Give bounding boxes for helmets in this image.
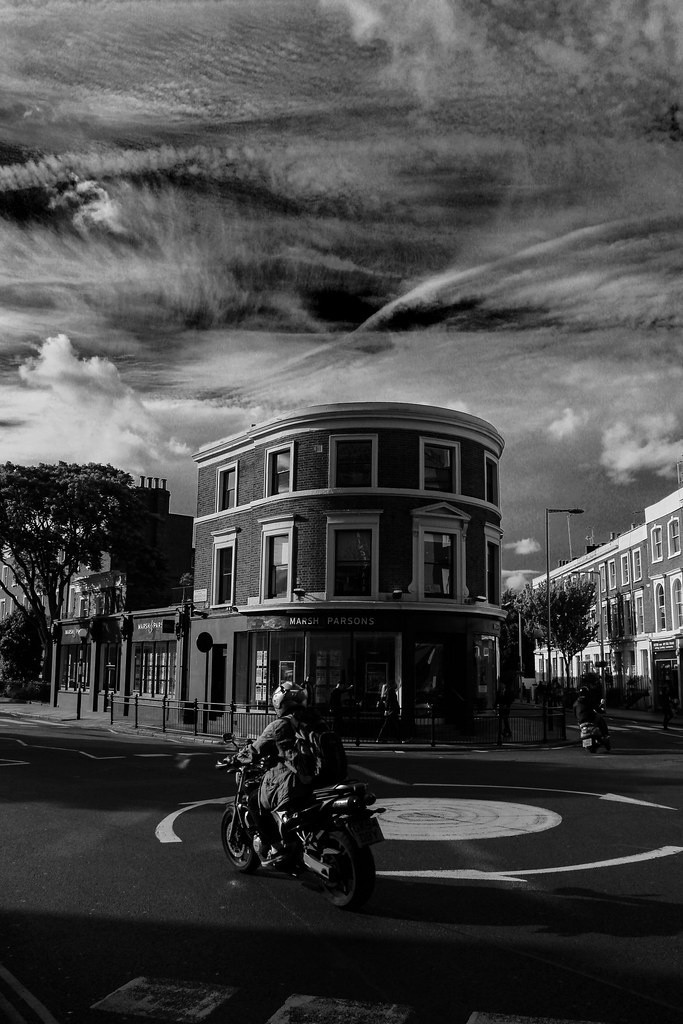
[579,686,589,695]
[272,681,306,718]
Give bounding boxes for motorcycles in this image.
[216,731,380,909]
[581,698,611,754]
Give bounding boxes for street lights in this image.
[542,501,612,709]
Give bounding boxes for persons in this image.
[327,676,572,744]
[572,687,610,738]
[660,685,676,730]
[217,680,349,867]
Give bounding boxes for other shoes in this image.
[402,736,413,744]
[261,850,284,865]
[605,734,611,740]
